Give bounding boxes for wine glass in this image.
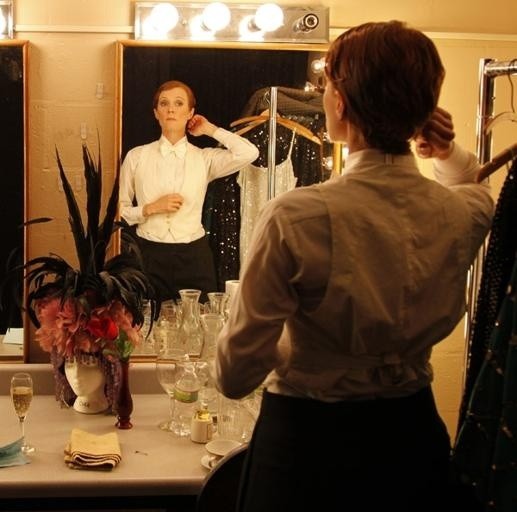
[7,373,35,455]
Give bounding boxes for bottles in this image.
[135,270,255,358]
[132,314,276,472]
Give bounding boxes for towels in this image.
[62,430,121,470]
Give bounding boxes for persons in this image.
[118,80,261,323]
[212,20,495,512]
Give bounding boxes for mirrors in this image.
[113,39,342,361]
[0,41,30,364]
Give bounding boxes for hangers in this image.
[462,57,517,376]
[228,93,322,142]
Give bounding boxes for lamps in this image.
[248,4,284,33]
[149,3,180,33]
[201,3,231,31]
